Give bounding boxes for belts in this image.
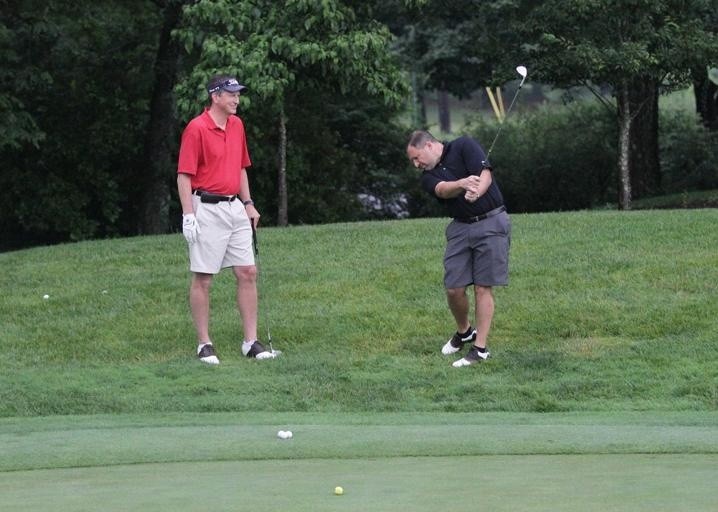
[457,205,507,224]
[192,189,237,201]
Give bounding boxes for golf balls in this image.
[335,486,343,495]
[278,430,293,440]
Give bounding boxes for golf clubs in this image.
[477,64,528,176]
[251,218,281,355]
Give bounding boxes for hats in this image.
[206,77,246,93]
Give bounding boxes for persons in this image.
[177,73,273,364]
[403,130,511,368]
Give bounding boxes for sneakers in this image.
[241,339,275,359]
[197,341,219,365]
[452,346,491,367]
[441,327,477,356]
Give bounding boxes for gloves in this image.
[182,213,202,245]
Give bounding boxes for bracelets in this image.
[244,200,254,206]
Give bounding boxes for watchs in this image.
[476,194,480,199]
